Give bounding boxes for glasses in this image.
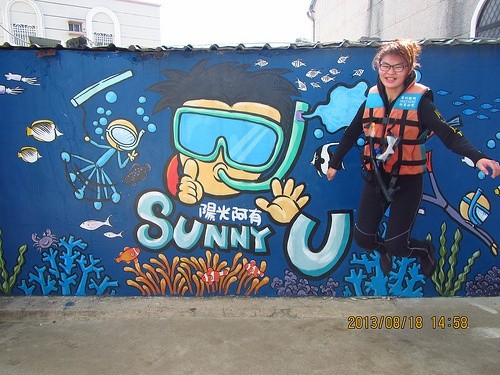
[378,61,410,73]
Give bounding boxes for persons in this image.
[325,41,500,277]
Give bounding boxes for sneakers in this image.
[378,238,393,276]
[419,241,436,276]
